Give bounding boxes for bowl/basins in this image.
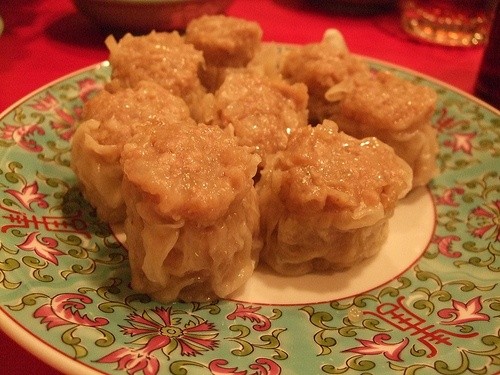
[71,0,236,36]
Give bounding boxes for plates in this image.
[0,43,500,374]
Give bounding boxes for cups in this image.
[397,0,497,49]
